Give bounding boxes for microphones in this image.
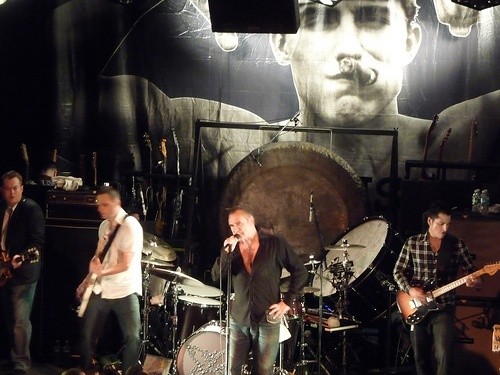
[323,257,337,272]
[290,110,301,121]
[309,194,314,222]
[225,234,240,249]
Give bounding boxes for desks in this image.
[40,187,102,240]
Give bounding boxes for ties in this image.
[9,209,14,220]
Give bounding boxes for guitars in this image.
[396,261,500,325]
[20,129,183,240]
[77,256,100,318]
[1,246,39,287]
[421,112,478,177]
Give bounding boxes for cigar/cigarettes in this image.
[339,57,377,85]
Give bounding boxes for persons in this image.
[56,0,500,185]
[0,171,45,375]
[155,215,167,234]
[76,190,143,375]
[212,207,308,375]
[393,208,481,375]
[36,162,57,185]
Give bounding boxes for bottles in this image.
[472,189,489,214]
[492,325,500,352]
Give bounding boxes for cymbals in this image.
[325,244,366,250]
[141,254,176,267]
[142,231,177,262]
[281,287,320,293]
[148,267,206,288]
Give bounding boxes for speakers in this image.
[38,215,122,362]
[446,214,500,375]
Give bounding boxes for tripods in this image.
[291,247,362,375]
[117,264,178,375]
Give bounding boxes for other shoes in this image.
[13,369,29,375]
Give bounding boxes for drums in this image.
[163,281,226,352]
[289,295,305,316]
[273,315,302,375]
[175,321,254,375]
[308,214,406,326]
